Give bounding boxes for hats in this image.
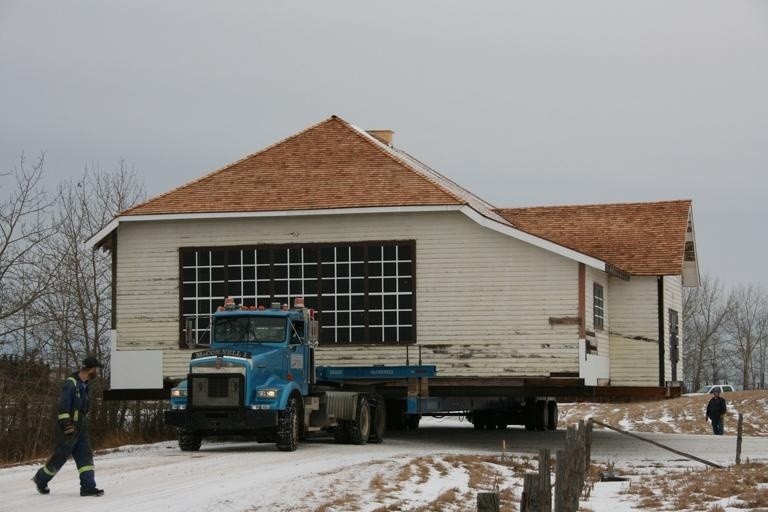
[81,357,104,368]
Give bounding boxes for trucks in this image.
[164,298,585,453]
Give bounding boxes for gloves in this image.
[63,420,74,437]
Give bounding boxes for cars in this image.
[679,385,734,397]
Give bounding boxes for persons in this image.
[32,356,105,497]
[705,390,726,435]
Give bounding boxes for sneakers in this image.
[33,475,49,494]
[80,488,104,496]
